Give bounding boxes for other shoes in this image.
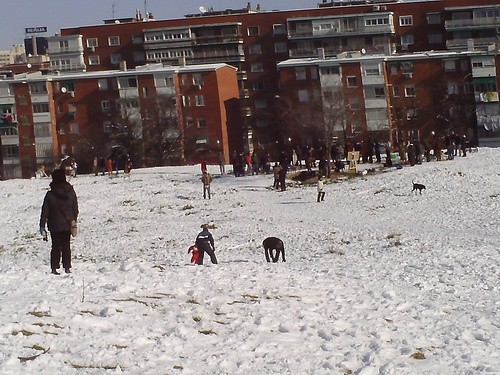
[52,271,60,275]
[65,268,72,273]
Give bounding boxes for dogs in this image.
[412,178,426,195]
[263,237,286,262]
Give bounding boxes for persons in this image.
[200,159,207,174]
[187,241,205,264]
[195,224,218,265]
[93,154,132,177]
[263,237,286,263]
[39,168,80,275]
[231,124,473,192]
[201,172,212,200]
[317,175,326,202]
[218,151,226,175]
[37,158,79,179]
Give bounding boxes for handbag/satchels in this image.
[71,219,77,237]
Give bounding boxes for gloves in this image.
[40,226,47,235]
